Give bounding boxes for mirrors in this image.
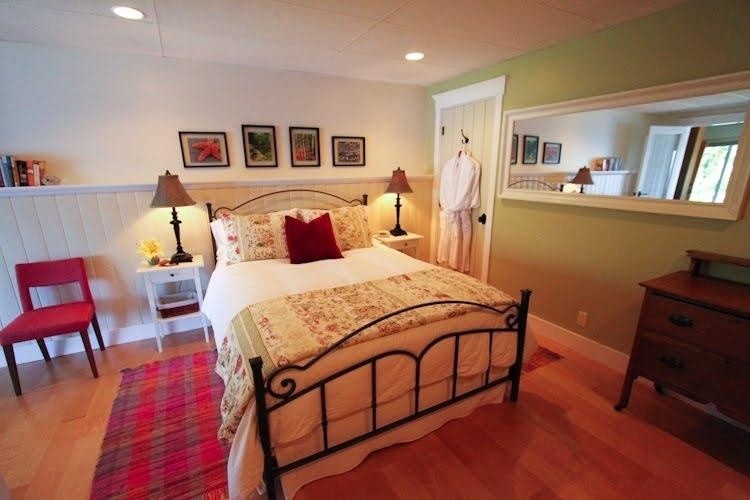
[497,70,750,222]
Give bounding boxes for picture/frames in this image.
[331,136,366,166]
[287,126,321,167]
[510,134,518,164]
[543,141,562,164]
[523,135,540,164]
[240,124,278,168]
[177,131,230,168]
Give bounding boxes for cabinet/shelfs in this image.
[614,250,750,427]
[508,170,640,196]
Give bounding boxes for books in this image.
[593,157,622,172]
[0,156,47,188]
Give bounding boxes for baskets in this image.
[155,291,199,318]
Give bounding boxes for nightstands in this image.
[372,233,423,259]
[136,255,209,354]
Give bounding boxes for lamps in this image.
[385,167,415,236]
[150,170,198,264]
[568,165,596,195]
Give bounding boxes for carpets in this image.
[89,344,567,500]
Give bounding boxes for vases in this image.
[146,255,159,264]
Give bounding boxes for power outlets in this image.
[576,310,589,329]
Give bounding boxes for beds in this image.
[204,189,533,500]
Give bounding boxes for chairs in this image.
[1,257,106,396]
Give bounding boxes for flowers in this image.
[136,239,161,262]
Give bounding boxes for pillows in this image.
[218,207,298,267]
[297,204,373,252]
[284,212,345,264]
